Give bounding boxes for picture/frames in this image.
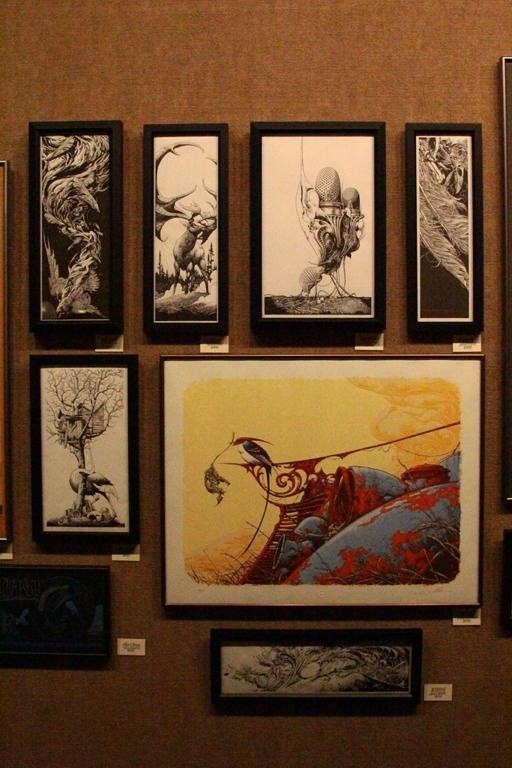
[0,563,111,664]
[143,124,229,334]
[159,354,484,607]
[501,56,512,505]
[29,353,140,545]
[405,122,483,338]
[0,160,11,543]
[501,528,512,637]
[250,120,386,332]
[210,629,423,706]
[29,120,123,335]
